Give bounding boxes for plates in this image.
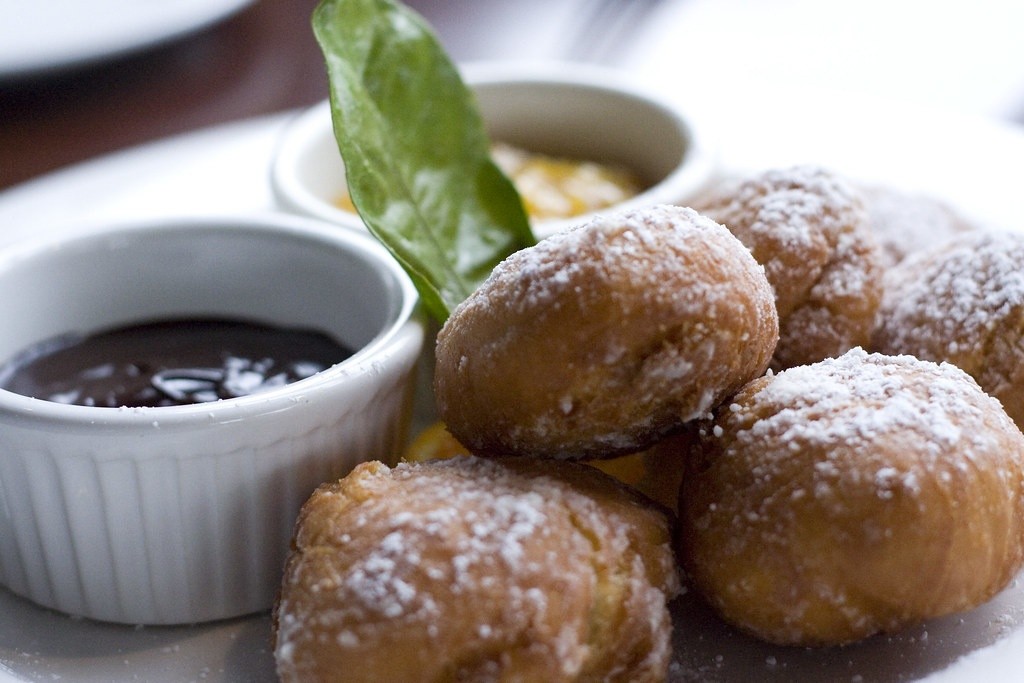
[0,105,1023,683]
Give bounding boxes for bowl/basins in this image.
[1,205,437,628]
[270,63,710,240]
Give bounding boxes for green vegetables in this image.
[314,2,535,323]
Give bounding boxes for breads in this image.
[275,165,1024,683]
